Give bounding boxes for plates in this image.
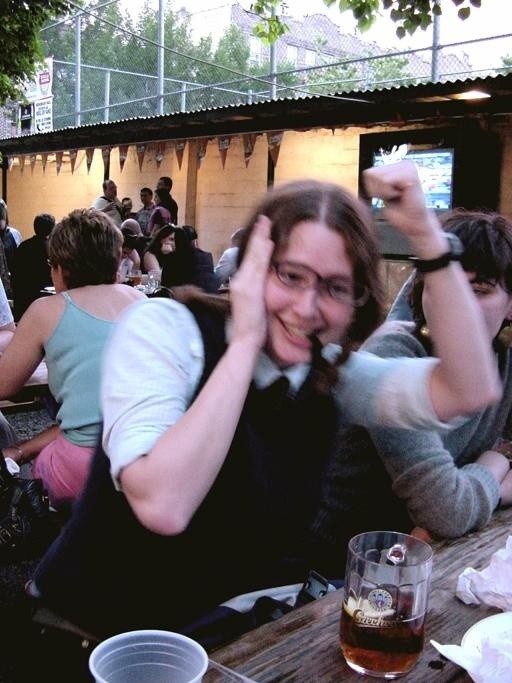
[458,611,512,682]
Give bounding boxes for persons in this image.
[214,229,244,283]
[0,198,23,293]
[95,177,178,235]
[10,155,504,676]
[10,213,55,302]
[307,209,512,570]
[118,207,220,292]
[2,207,149,505]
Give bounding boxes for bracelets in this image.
[413,233,461,271]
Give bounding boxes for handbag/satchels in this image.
[0,477,61,564]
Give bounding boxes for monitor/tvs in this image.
[369,148,455,220]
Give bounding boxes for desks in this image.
[203,509,511,683]
[1,355,49,415]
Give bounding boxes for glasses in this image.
[272,257,369,305]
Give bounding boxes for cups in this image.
[339,529,436,677]
[89,628,209,683]
[129,271,162,295]
